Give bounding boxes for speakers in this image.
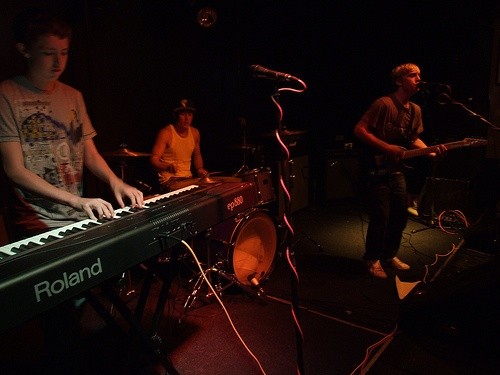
[268,155,308,214]
[426,176,474,223]
[324,154,363,200]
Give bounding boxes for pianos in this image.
[0,183,259,375]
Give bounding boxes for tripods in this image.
[181,230,243,310]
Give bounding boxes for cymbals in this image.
[99,148,151,156]
[169,173,242,191]
[263,129,307,135]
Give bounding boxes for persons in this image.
[0,19,144,375]
[149,98,210,192]
[353,64,448,280]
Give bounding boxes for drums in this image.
[205,206,279,293]
[238,166,276,205]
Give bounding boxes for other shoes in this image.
[390,257,410,270]
[366,258,388,279]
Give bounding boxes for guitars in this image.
[372,134,489,189]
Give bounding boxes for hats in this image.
[169,95,197,114]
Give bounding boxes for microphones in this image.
[248,275,266,294]
[419,81,448,89]
[250,64,297,83]
